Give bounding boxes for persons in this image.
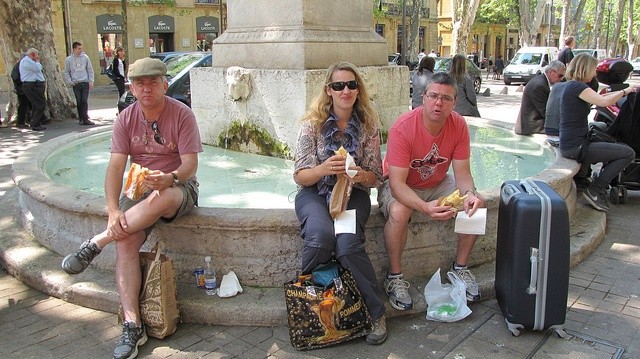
[292,61,389,346]
[112,46,126,99]
[495,54,505,80]
[11,52,51,129]
[105,40,110,51]
[448,54,481,117]
[487,54,493,72]
[558,35,576,66]
[428,50,437,57]
[559,52,636,212]
[63,42,95,125]
[515,61,566,135]
[418,48,426,64]
[19,48,46,131]
[412,56,436,110]
[61,56,203,358]
[105,45,112,61]
[377,72,486,312]
[544,82,568,135]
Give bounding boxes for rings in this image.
[332,167,333,170]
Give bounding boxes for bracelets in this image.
[464,190,475,195]
[622,89,626,97]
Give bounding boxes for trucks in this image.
[571,48,606,61]
[503,47,560,85]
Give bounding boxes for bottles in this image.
[203,255,217,295]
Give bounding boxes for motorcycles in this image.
[594,57,640,127]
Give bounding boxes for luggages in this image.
[495,180,570,338]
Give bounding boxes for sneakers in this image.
[366,315,387,344]
[113,320,147,359]
[446,261,481,301]
[582,184,610,212]
[84,120,95,125]
[62,238,101,274]
[383,270,414,311]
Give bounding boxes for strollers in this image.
[591,89,640,205]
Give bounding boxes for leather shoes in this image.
[32,125,46,129]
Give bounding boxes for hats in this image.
[127,57,167,78]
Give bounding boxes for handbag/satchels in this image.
[284,252,375,351]
[118,242,177,339]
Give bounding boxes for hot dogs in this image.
[334,144,349,157]
[440,190,467,210]
[141,167,159,178]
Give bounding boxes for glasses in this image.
[553,69,564,78]
[329,81,358,91]
[152,120,164,144]
[425,94,453,103]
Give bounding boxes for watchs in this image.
[170,172,179,188]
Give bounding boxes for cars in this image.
[118,51,212,114]
[149,52,183,63]
[467,55,488,69]
[630,57,640,75]
[388,52,401,66]
[410,56,483,97]
